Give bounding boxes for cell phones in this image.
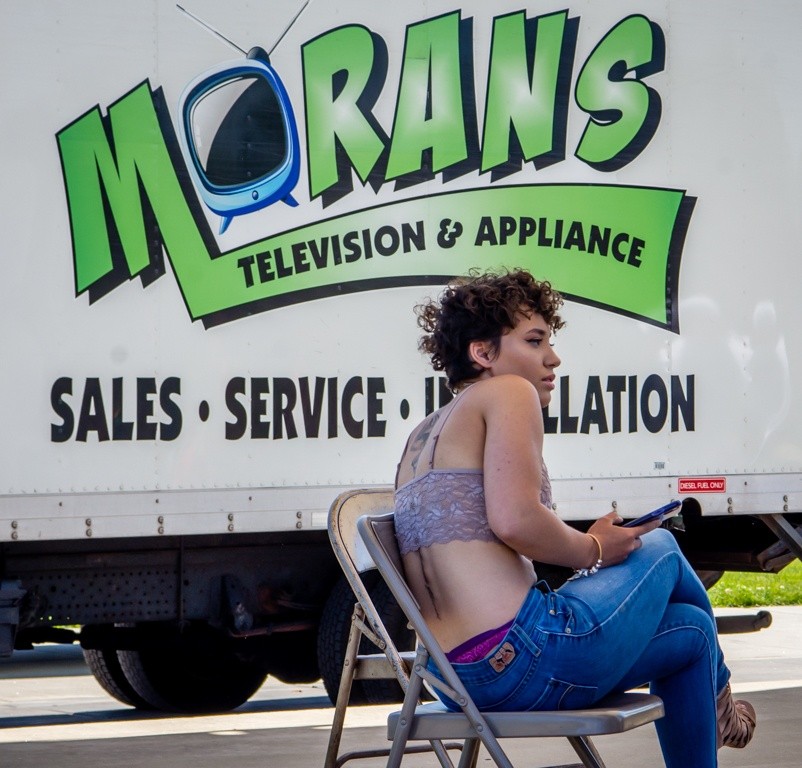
[623,500,682,528]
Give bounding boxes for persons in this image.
[392,265,756,768]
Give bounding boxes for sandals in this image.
[717,682,756,752]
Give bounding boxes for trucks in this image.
[1,0,802,720]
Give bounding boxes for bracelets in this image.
[573,533,603,577]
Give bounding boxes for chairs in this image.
[356,511,665,768]
[324,490,456,768]
